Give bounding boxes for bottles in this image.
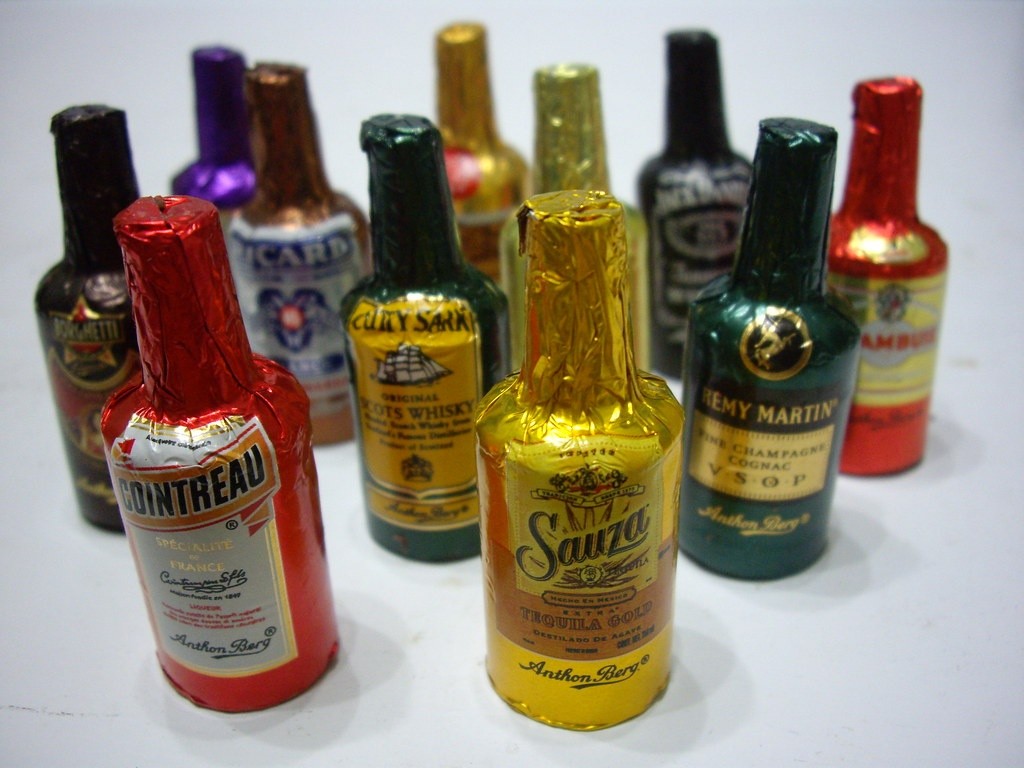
[227,61,372,448]
[432,25,526,291]
[472,190,685,732]
[100,195,337,713]
[677,118,862,581]
[171,47,257,233]
[35,103,143,534]
[338,112,509,566]
[635,30,754,382]
[823,79,948,477]
[497,62,649,377]
